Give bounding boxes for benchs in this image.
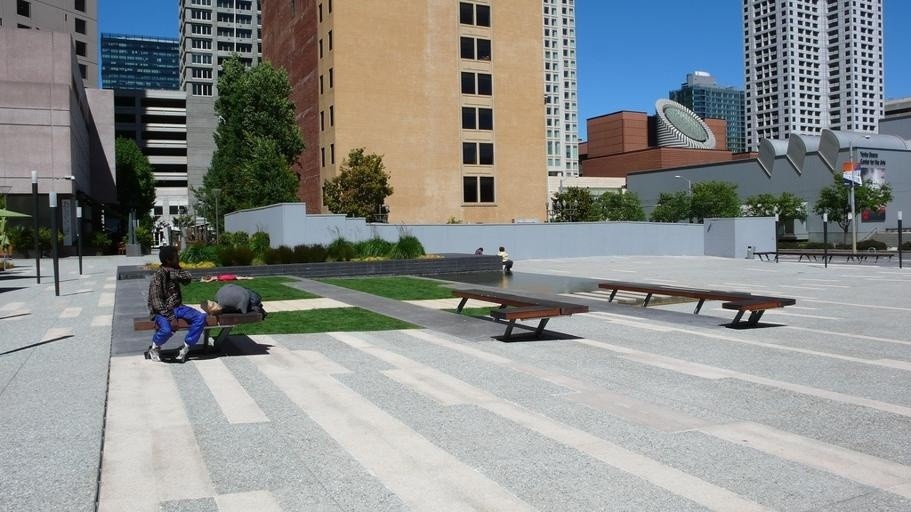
[452,289,589,341]
[133,310,263,359]
[599,282,796,326]
[753,251,899,264]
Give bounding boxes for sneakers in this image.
[175,347,189,363]
[148,346,161,362]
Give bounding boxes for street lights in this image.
[76,207,83,275]
[49,191,59,297]
[31,171,40,284]
[849,135,871,253]
[824,214,827,268]
[676,175,692,194]
[898,211,902,268]
